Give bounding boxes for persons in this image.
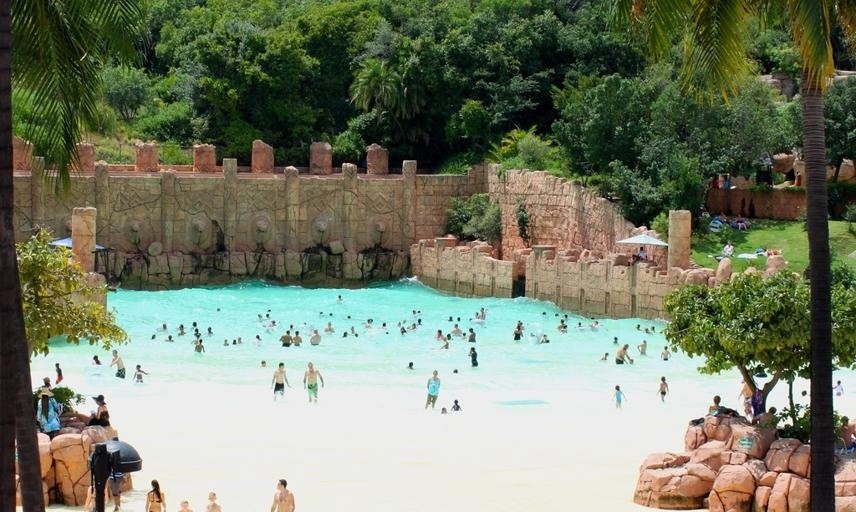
[637,246,647,260]
[708,171,802,257]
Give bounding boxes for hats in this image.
[38,387,55,398]
[92,395,107,405]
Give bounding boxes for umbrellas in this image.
[51,237,104,251]
[618,234,668,251]
